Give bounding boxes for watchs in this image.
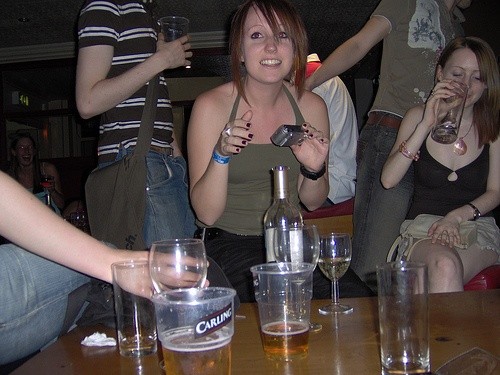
[465,202,481,221]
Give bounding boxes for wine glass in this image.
[315,232,355,315]
[273,224,323,334]
[147,238,207,370]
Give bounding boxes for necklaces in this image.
[452,118,477,158]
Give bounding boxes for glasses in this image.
[16,144,34,151]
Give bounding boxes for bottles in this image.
[45,194,55,212]
[262,165,307,322]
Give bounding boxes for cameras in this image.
[270,125,307,147]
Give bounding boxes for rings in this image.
[224,127,232,136]
[431,88,436,95]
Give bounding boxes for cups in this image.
[111,259,158,358]
[157,15,190,43]
[151,287,236,375]
[376,262,432,375]
[431,80,468,144]
[70,209,88,230]
[250,262,315,363]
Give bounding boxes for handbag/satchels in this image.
[84,154,147,252]
[57,255,241,338]
[386,213,477,263]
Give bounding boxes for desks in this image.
[6,287,500,375]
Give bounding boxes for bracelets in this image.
[398,138,421,162]
[209,145,232,165]
[299,161,327,181]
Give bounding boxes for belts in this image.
[150,144,174,157]
[194,229,265,241]
[366,112,401,128]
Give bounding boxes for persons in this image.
[284,50,360,206]
[4,132,66,212]
[303,0,471,295]
[186,0,379,303]
[0,170,210,366]
[73,0,200,253]
[380,35,500,295]
